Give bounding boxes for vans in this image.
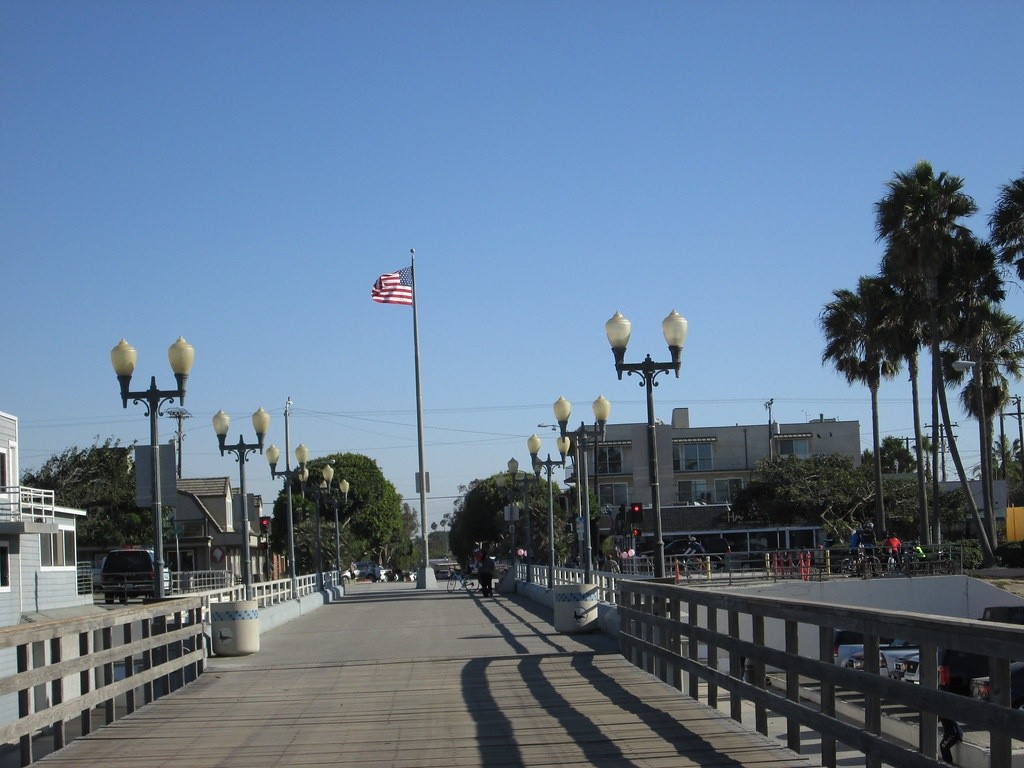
[341,560,381,583]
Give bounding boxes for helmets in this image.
[889,533,897,538]
[867,522,875,530]
[856,526,864,532]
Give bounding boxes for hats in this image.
[607,554,612,559]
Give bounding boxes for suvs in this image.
[101,548,174,605]
[637,537,732,575]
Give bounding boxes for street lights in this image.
[553,394,611,587]
[507,457,544,581]
[299,465,334,593]
[604,310,689,683]
[527,433,571,588]
[110,335,196,706]
[266,442,309,600]
[213,405,270,600]
[319,478,351,588]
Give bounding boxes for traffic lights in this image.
[300,504,310,522]
[631,502,644,524]
[259,516,270,535]
[633,528,642,536]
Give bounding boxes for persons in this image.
[263,559,274,581]
[348,562,356,586]
[609,545,621,557]
[913,541,926,561]
[884,533,905,567]
[938,717,963,765]
[832,522,877,577]
[476,550,494,597]
[701,493,711,503]
[603,555,621,573]
[684,537,705,562]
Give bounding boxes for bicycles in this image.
[840,548,942,580]
[936,546,956,575]
[446,566,480,593]
[672,554,715,577]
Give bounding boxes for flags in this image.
[371,265,412,306]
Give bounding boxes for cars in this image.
[437,569,452,581]
[378,567,418,582]
[831,628,1024,711]
